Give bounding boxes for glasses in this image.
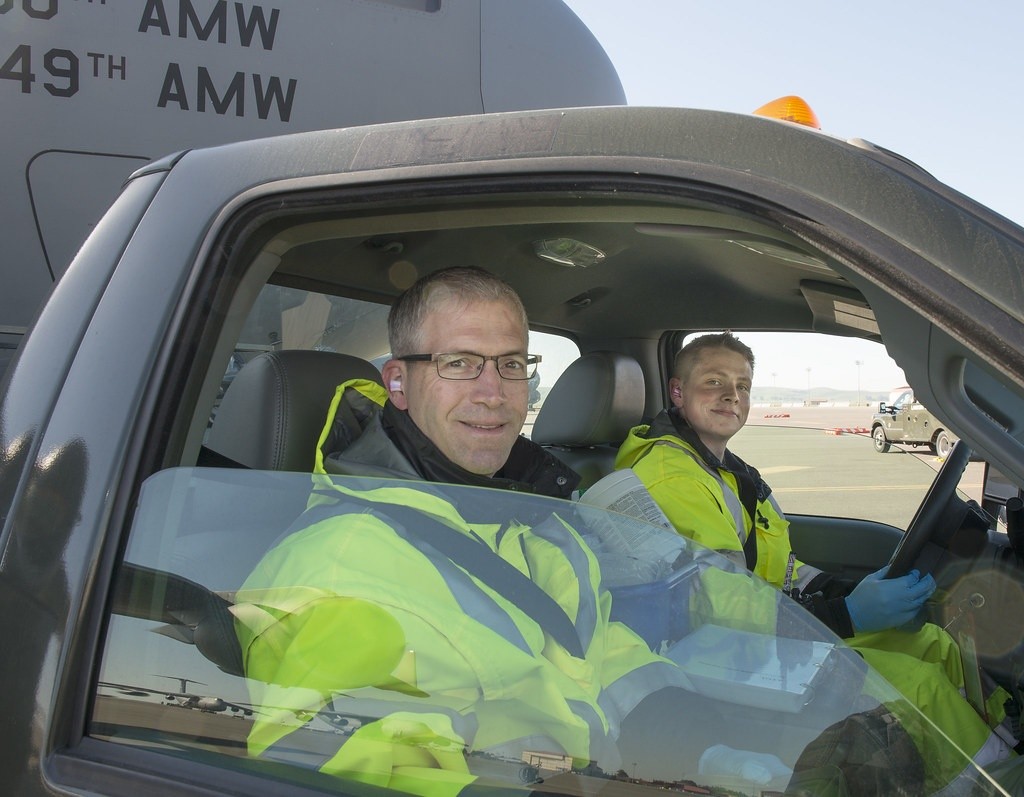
[396,352,542,380]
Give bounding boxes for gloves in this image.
[698,744,794,785]
[845,566,937,633]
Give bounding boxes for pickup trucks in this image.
[870,386,961,461]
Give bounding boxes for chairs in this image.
[531,352,645,490]
[205,350,385,473]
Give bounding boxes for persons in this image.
[229,265,794,797]
[610,329,1024,796]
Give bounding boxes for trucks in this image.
[1,0,1024,796]
